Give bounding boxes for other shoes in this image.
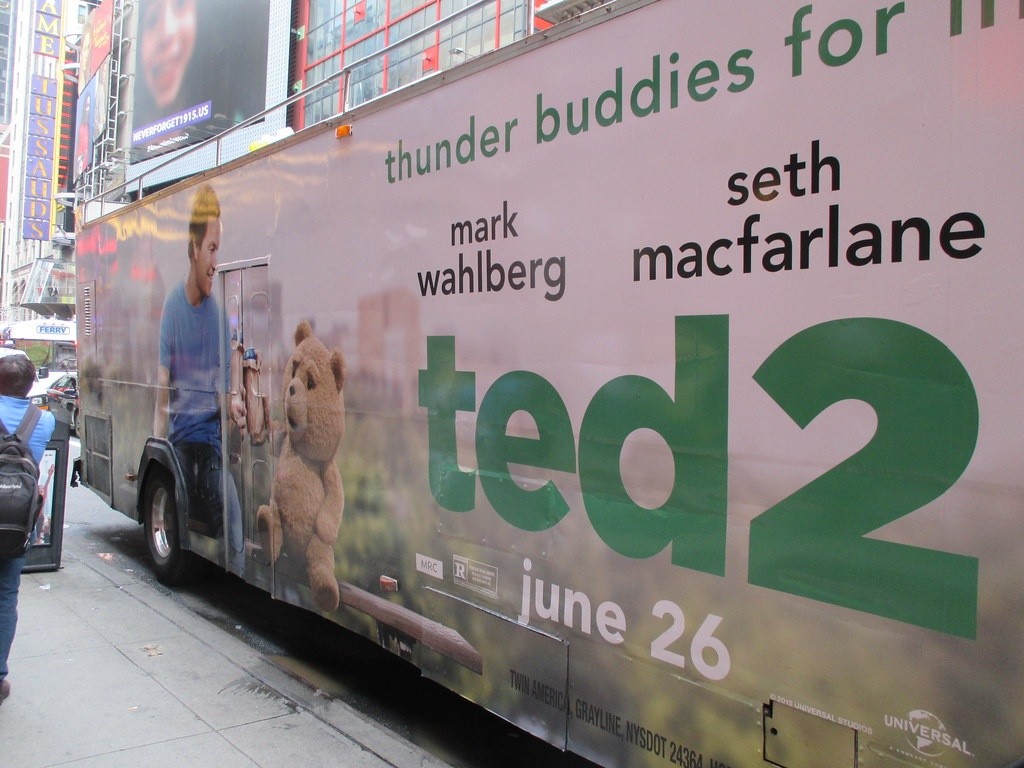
[0,679,10,704]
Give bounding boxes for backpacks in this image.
[0,397,43,562]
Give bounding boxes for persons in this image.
[154,185,247,580]
[0,355,55,704]
[134,0,220,162]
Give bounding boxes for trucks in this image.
[70,0,1023,768]
[0,312,78,411]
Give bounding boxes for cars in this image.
[46,374,81,439]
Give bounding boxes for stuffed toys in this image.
[257,320,347,613]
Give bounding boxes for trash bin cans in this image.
[20,394,72,574]
[68,359,77,370]
